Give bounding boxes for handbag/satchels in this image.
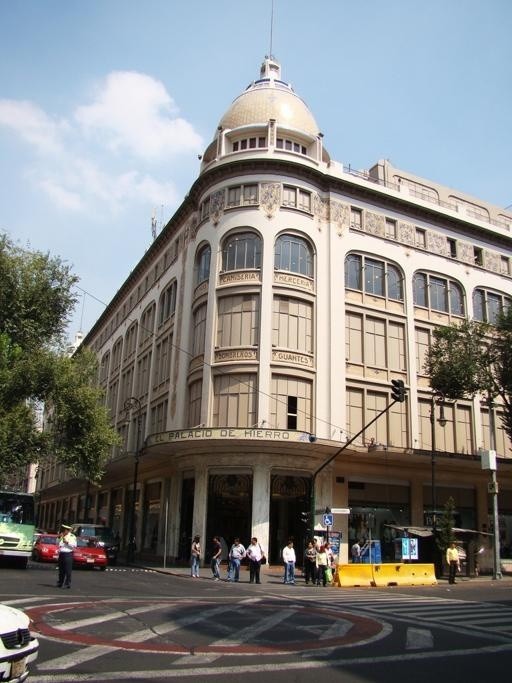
[324,568,335,584]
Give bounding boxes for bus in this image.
[1,489,40,569]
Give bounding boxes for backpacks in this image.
[261,556,267,566]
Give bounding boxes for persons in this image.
[302,537,338,587]
[350,538,360,563]
[191,534,201,578]
[282,540,295,584]
[225,536,245,583]
[207,536,222,581]
[445,541,460,584]
[56,523,78,590]
[246,537,265,584]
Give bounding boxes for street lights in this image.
[35,465,45,528]
[479,390,503,580]
[430,391,449,579]
[121,397,141,562]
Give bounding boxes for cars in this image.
[1,603,40,682]
[33,523,119,571]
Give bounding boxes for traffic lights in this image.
[390,378,403,402]
[300,511,310,530]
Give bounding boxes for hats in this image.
[61,523,73,531]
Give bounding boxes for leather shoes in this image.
[447,581,458,584]
[192,575,200,577]
